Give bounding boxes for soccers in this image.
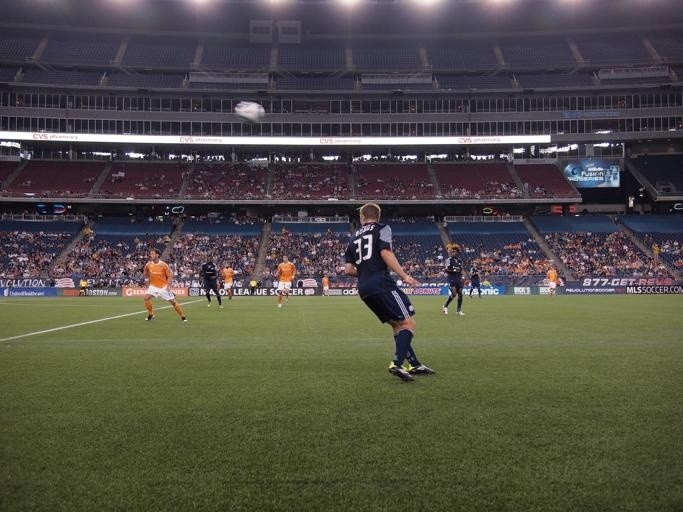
[234,100,266,123]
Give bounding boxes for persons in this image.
[79,279,88,296]
[143,249,187,322]
[321,275,329,297]
[248,278,257,296]
[546,266,557,295]
[221,264,235,300]
[199,254,224,309]
[345,202,436,382]
[273,255,296,308]
[442,248,465,316]
[469,262,482,299]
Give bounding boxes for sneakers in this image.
[145,314,155,321]
[442,305,448,314]
[208,299,212,307]
[277,304,282,308]
[219,305,223,308]
[182,317,188,322]
[286,300,288,303]
[389,360,435,381]
[456,311,465,315]
